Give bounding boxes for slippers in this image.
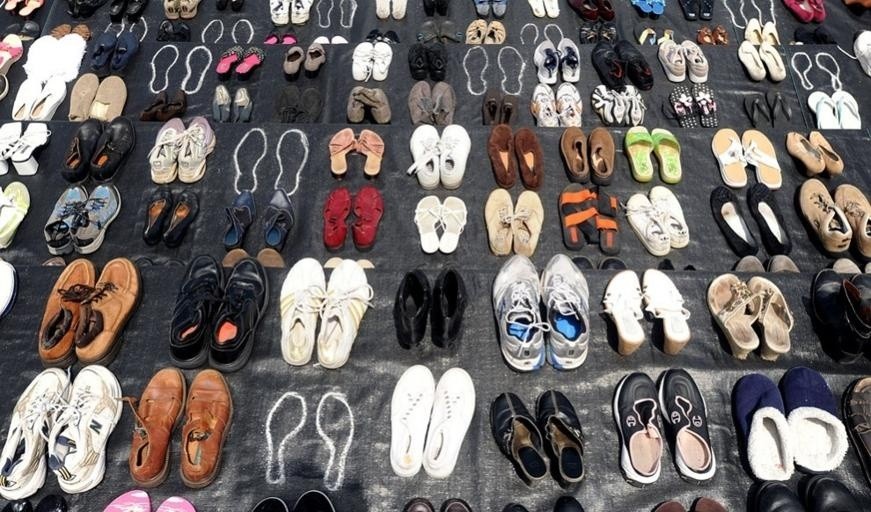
[216,45,244,79]
[235,46,264,79]
[18,0,44,21]
[465,19,487,45]
[669,84,699,127]
[328,127,356,176]
[383,30,400,44]
[580,21,598,43]
[366,28,382,44]
[741,129,783,190]
[357,129,384,179]
[711,128,748,188]
[691,83,720,128]
[18,20,39,41]
[0,33,23,75]
[270,0,290,26]
[11,80,40,122]
[30,74,67,122]
[290,0,313,25]
[474,0,490,18]
[416,21,439,43]
[4,0,22,15]
[630,0,652,18]
[173,22,190,42]
[633,21,657,45]
[700,0,714,20]
[491,0,507,19]
[651,128,682,184]
[108,0,128,23]
[413,194,441,256]
[438,196,468,254]
[778,366,848,476]
[679,0,700,20]
[0,23,20,40]
[156,19,173,42]
[624,125,654,183]
[263,188,294,251]
[731,373,795,484]
[440,20,462,44]
[646,0,666,20]
[832,90,862,129]
[126,0,147,20]
[808,90,841,129]
[766,88,792,128]
[744,93,772,128]
[598,21,618,44]
[68,73,99,121]
[89,75,127,122]
[558,182,620,255]
[483,20,506,44]
[657,24,675,45]
[222,190,255,251]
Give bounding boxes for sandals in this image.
[72,24,91,41]
[4,122,51,176]
[372,42,392,82]
[843,377,871,480]
[0,122,21,176]
[51,23,71,39]
[710,186,759,257]
[351,42,374,83]
[746,182,793,254]
[352,185,385,253]
[322,187,352,254]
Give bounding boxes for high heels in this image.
[536,390,585,490]
[283,46,305,82]
[786,131,825,177]
[138,91,168,122]
[109,31,139,78]
[642,267,691,356]
[747,276,794,362]
[490,392,548,489]
[155,89,186,120]
[809,131,844,179]
[280,26,297,45]
[89,30,116,77]
[707,273,763,360]
[303,43,325,79]
[602,270,643,356]
[263,27,281,45]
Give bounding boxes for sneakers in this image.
[212,85,231,122]
[163,0,180,20]
[178,116,216,184]
[428,42,447,82]
[834,183,871,260]
[533,39,559,85]
[0,181,30,250]
[388,364,435,477]
[279,257,326,366]
[483,88,501,124]
[439,124,471,190]
[626,193,671,257]
[619,84,646,126]
[179,0,201,19]
[271,85,301,124]
[783,0,813,24]
[543,0,560,18]
[528,0,546,17]
[431,82,456,125]
[74,256,142,367]
[591,39,628,87]
[38,258,99,370]
[614,40,654,91]
[561,125,589,184]
[316,259,370,371]
[422,367,476,480]
[656,366,716,485]
[587,127,614,186]
[500,95,518,125]
[539,254,590,370]
[410,124,440,190]
[555,81,583,127]
[0,366,71,502]
[407,80,433,124]
[232,87,252,124]
[531,82,559,128]
[853,30,871,77]
[658,39,686,83]
[649,186,690,249]
[808,0,826,24]
[296,88,323,123]
[612,371,664,489]
[514,128,544,191]
[591,84,625,126]
[557,38,581,83]
[758,41,787,82]
[492,253,546,372]
[513,191,544,256]
[680,40,709,84]
[44,182,89,256]
[799,178,853,258]
[47,363,122,495]
[484,188,514,257]
[69,182,122,254]
[737,40,766,82]
[408,43,427,79]
[149,117,186,184]
[488,123,515,188]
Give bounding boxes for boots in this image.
[348,86,380,124]
[812,268,871,365]
[394,268,430,349]
[851,273,871,304]
[354,87,392,123]
[431,266,468,350]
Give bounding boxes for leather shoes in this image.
[697,23,715,45]
[2,498,32,512]
[572,256,597,270]
[439,498,471,512]
[231,0,244,12]
[115,367,187,488]
[757,480,807,512]
[592,0,616,23]
[142,182,173,244]
[762,21,781,45]
[168,253,226,369]
[403,498,434,512]
[713,24,729,46]
[567,1,599,22]
[179,368,233,489]
[60,117,105,185]
[216,0,228,10]
[89,115,136,187]
[161,185,200,249]
[599,257,628,270]
[67,0,80,17]
[804,472,859,512]
[744,18,764,45]
[33,494,67,512]
[77,0,106,17]
[208,255,269,373]
